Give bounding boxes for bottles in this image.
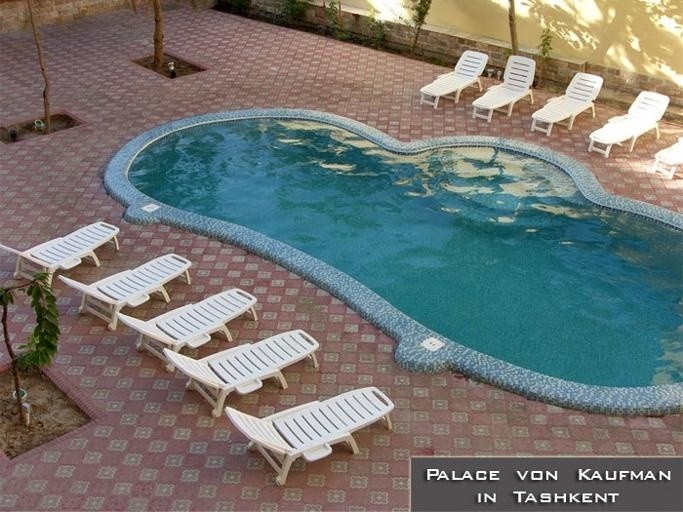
[7,128,16,142]
[167,62,176,79]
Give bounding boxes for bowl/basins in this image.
[34,119,43,130]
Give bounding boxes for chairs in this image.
[530,71,605,137]
[652,140,683,180]
[115,288,258,374]
[471,55,537,124]
[419,49,489,110]
[57,253,192,332]
[162,329,320,419]
[223,385,395,487]
[0,221,120,290]
[588,91,671,159]
